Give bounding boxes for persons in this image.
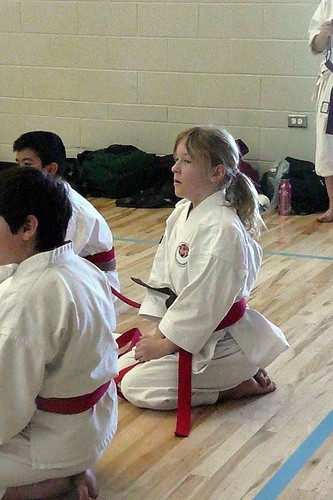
[114,125,289,412]
[307,0,333,223]
[0,130,120,500]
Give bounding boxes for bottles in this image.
[278,179,292,216]
[266,168,277,180]
